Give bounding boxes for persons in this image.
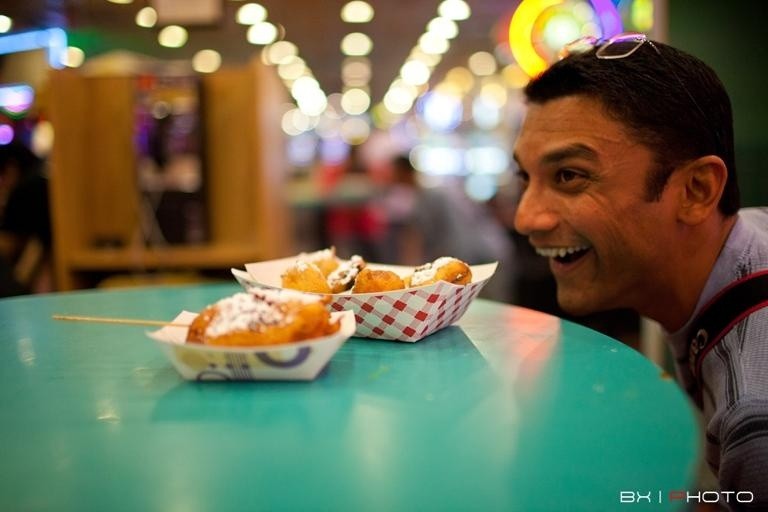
[1,118,54,297]
[386,156,516,303]
[511,36,766,511]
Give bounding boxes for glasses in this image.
[564,31,706,120]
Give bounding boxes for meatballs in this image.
[186,290,341,346]
[283,248,472,292]
